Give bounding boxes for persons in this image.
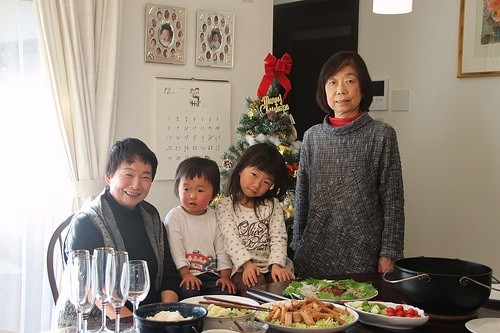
[51,137,180,330]
[164,144,295,298]
[149,9,231,62]
[290,52,405,279]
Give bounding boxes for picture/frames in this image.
[144,3,188,64]
[456,0,500,79]
[196,9,235,68]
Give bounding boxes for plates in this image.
[254,299,359,333]
[343,301,430,330]
[291,279,379,305]
[465,318,500,333]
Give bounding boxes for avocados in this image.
[354,300,388,315]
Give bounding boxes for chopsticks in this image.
[198,296,274,312]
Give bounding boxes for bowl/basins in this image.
[178,294,260,330]
[134,303,208,333]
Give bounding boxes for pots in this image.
[382,256,500,316]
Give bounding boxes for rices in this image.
[146,310,194,321]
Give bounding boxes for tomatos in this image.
[385,305,422,317]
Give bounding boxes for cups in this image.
[230,321,269,333]
[200,329,239,333]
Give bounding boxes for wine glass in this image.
[64,248,150,333]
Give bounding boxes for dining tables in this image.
[78,273,477,333]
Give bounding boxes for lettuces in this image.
[283,277,377,300]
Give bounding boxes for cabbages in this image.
[260,309,355,329]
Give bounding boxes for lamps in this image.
[371,0,412,15]
